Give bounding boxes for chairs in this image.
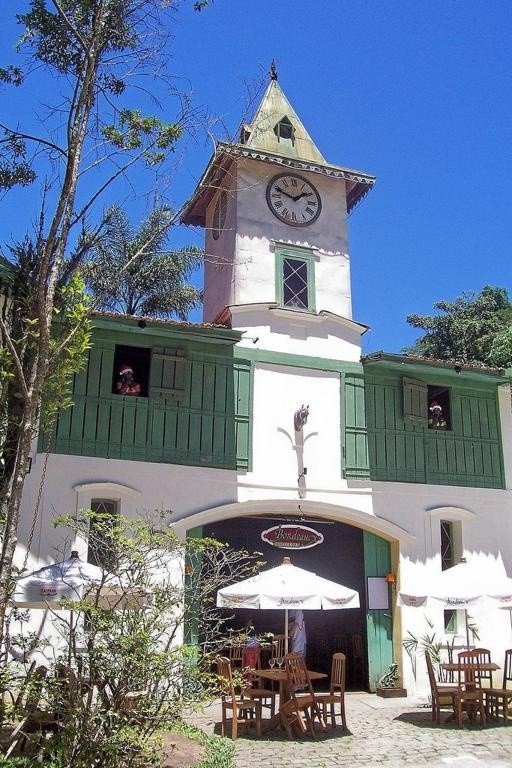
[425,647,512,729]
[216,632,368,741]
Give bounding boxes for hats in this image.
[119,365,134,375]
[429,400,441,411]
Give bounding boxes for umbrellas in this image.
[10,552,157,670]
[217,557,361,670]
[396,559,512,681]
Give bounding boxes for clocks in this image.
[266,172,322,228]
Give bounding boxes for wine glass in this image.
[268,659,275,672]
[276,658,283,673]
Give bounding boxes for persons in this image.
[427,401,447,430]
[115,365,141,397]
[285,609,310,692]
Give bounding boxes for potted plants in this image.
[376,662,407,699]
